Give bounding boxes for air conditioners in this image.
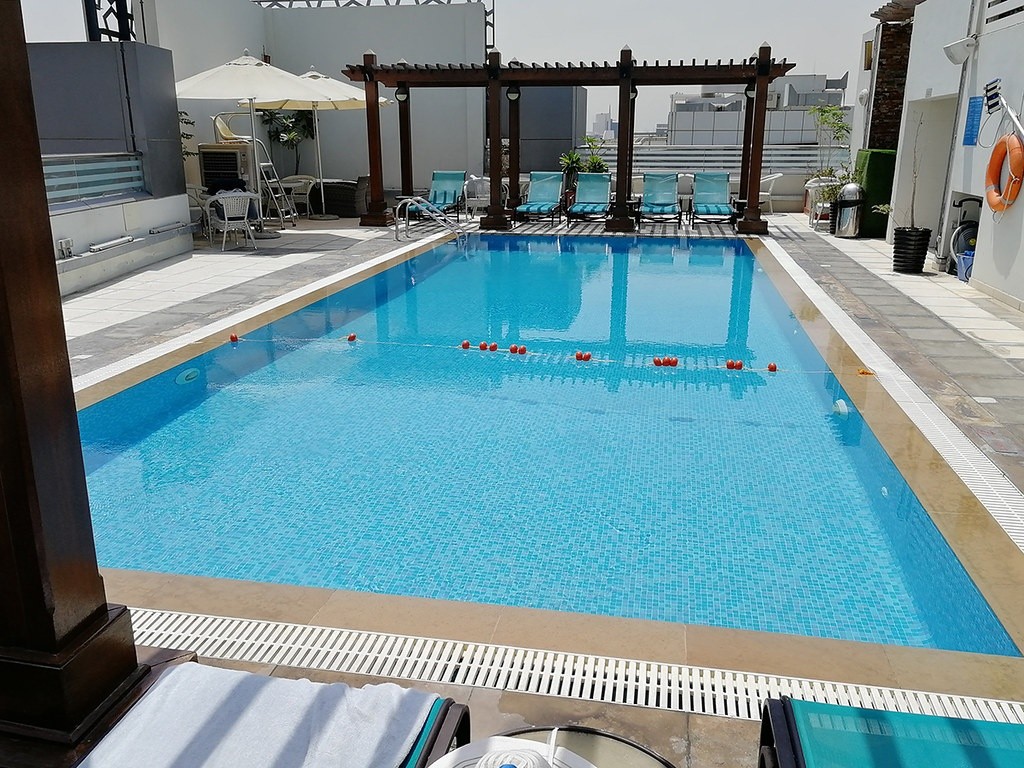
[766,93,777,108]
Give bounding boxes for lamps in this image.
[745,84,755,98]
[395,86,408,101]
[506,86,521,101]
[631,85,638,100]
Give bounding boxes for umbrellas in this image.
[174,47,357,240]
[238,64,395,220]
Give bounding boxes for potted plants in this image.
[802,104,852,220]
[558,136,608,208]
[871,112,933,273]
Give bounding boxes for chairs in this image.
[409,171,467,222]
[686,171,739,230]
[566,172,611,228]
[501,170,566,227]
[639,172,683,230]
[464,177,490,223]
[759,172,783,215]
[188,173,317,251]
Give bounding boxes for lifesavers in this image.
[985,131,1024,211]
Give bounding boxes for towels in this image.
[76,661,441,768]
